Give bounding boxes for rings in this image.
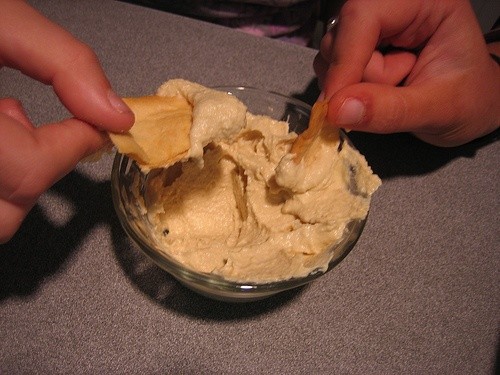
[324,14,339,33]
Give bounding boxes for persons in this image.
[0,2,133,247]
[314,1,500,148]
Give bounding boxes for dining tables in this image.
[0,0,500,375]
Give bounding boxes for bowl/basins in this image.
[110,83,371,301]
